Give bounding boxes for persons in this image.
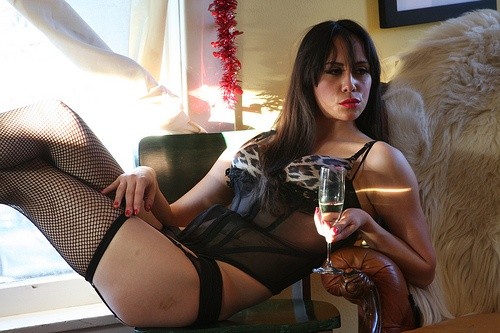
[0,19,437,330]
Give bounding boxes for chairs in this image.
[132,131,340,333]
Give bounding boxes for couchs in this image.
[322,244,500,333]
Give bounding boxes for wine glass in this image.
[312,166,346,275]
